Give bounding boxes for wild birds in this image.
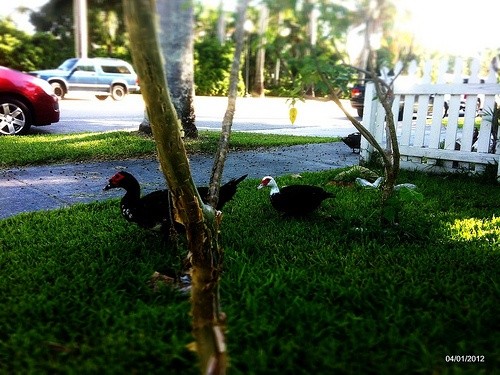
[342,131,361,154]
[102,171,249,235]
[257,176,337,214]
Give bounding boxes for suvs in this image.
[23,56,141,102]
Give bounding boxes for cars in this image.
[443,75,489,115]
[0,66,60,137]
[348,79,448,122]
